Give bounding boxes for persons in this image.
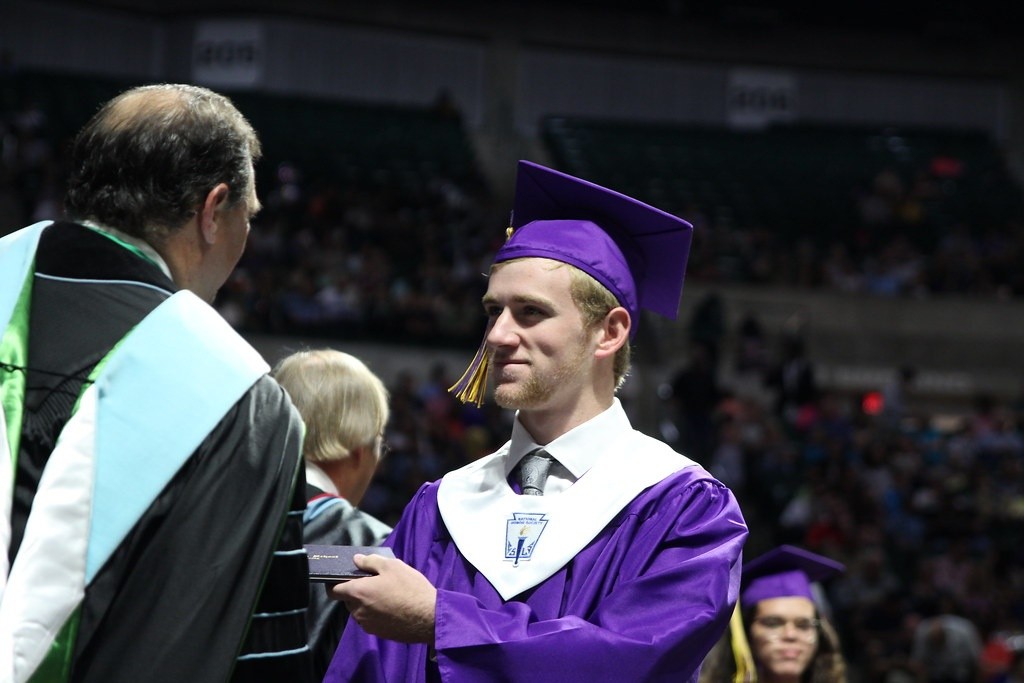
[698,545,849,683]
[0,83,1024,683]
[323,160,749,682]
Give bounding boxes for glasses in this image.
[379,433,391,461]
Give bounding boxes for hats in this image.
[740,545,847,619]
[446,159,696,409]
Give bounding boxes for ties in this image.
[519,448,555,496]
